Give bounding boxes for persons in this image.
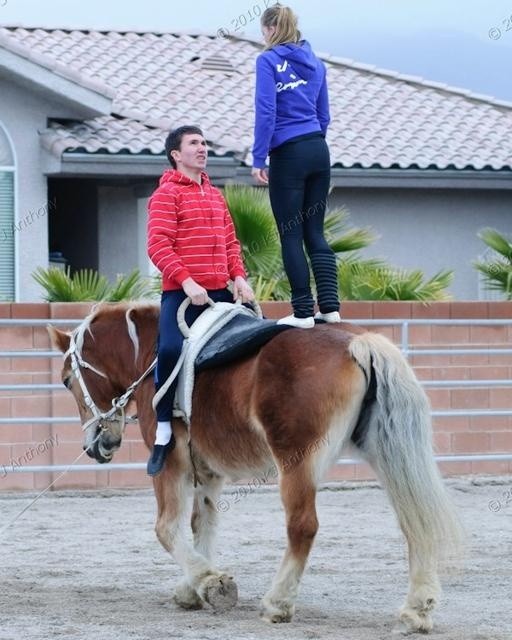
[251,1,343,328]
[145,125,263,478]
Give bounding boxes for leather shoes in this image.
[148,431,176,475]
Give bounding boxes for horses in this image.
[47,300,462,635]
